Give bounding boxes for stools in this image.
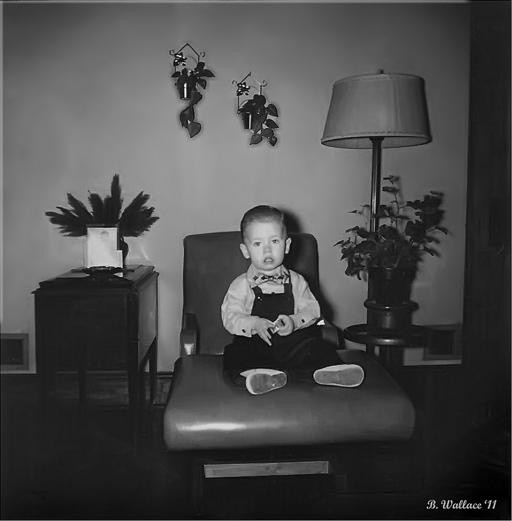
[166,347,417,498]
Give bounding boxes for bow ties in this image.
[252,270,289,287]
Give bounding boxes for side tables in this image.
[27,264,159,447]
[340,323,433,379]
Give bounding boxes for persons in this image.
[221,204,364,395]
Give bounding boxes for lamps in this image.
[321,68,434,356]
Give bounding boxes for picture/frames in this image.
[85,224,120,268]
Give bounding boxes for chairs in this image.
[179,231,337,356]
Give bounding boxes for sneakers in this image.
[244,368,288,395]
[313,364,364,388]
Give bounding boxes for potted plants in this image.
[47,172,162,263]
[333,166,448,334]
[171,61,216,140]
[238,93,280,146]
